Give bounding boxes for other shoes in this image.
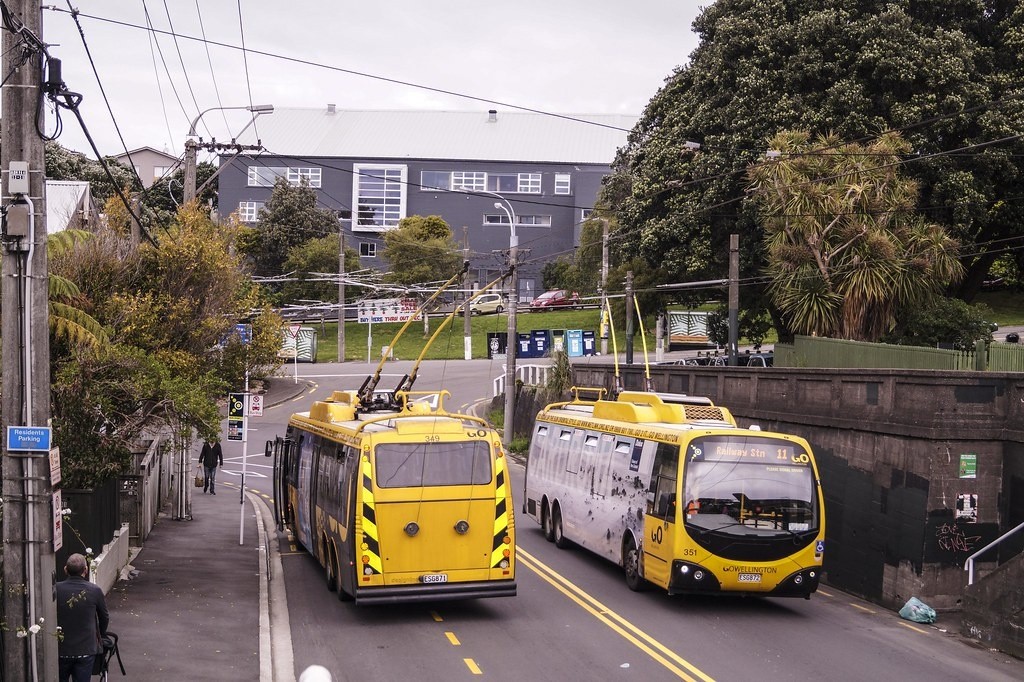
[210,491,215,495]
[204,487,207,492]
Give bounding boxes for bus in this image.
[265,257,517,604]
[522,294,836,603]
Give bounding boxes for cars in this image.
[530,289,580,311]
[456,294,505,318]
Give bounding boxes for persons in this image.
[669,487,702,518]
[298,460,311,550]
[56,554,109,682]
[197,434,223,495]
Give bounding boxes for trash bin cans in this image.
[487,328,596,359]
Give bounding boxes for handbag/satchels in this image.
[195,466,203,487]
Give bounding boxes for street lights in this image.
[181,102,275,208]
[460,185,515,452]
